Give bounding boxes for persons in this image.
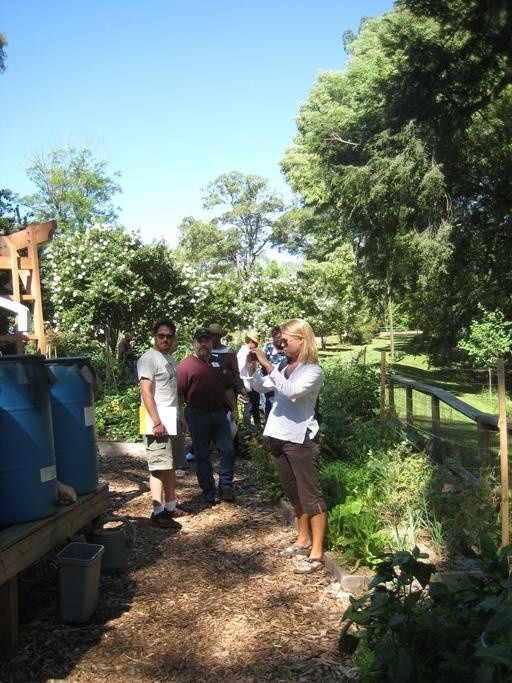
[176,328,241,505]
[257,323,286,419]
[182,320,239,461]
[245,317,329,575]
[136,316,197,528]
[234,330,262,436]
[116,330,137,377]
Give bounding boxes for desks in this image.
[2,482,110,646]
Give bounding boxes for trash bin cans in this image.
[56,542,105,623]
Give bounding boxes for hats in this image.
[193,328,211,339]
[246,329,261,343]
[205,323,224,337]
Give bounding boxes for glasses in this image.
[281,335,300,346]
[155,333,174,339]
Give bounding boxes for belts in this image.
[202,407,218,411]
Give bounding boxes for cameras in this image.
[249,351,258,361]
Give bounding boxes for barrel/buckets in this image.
[1,354,59,523]
[44,355,99,496]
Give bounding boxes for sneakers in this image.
[293,557,324,574]
[204,490,215,502]
[279,541,313,558]
[148,509,182,529]
[219,488,234,501]
[165,507,191,518]
